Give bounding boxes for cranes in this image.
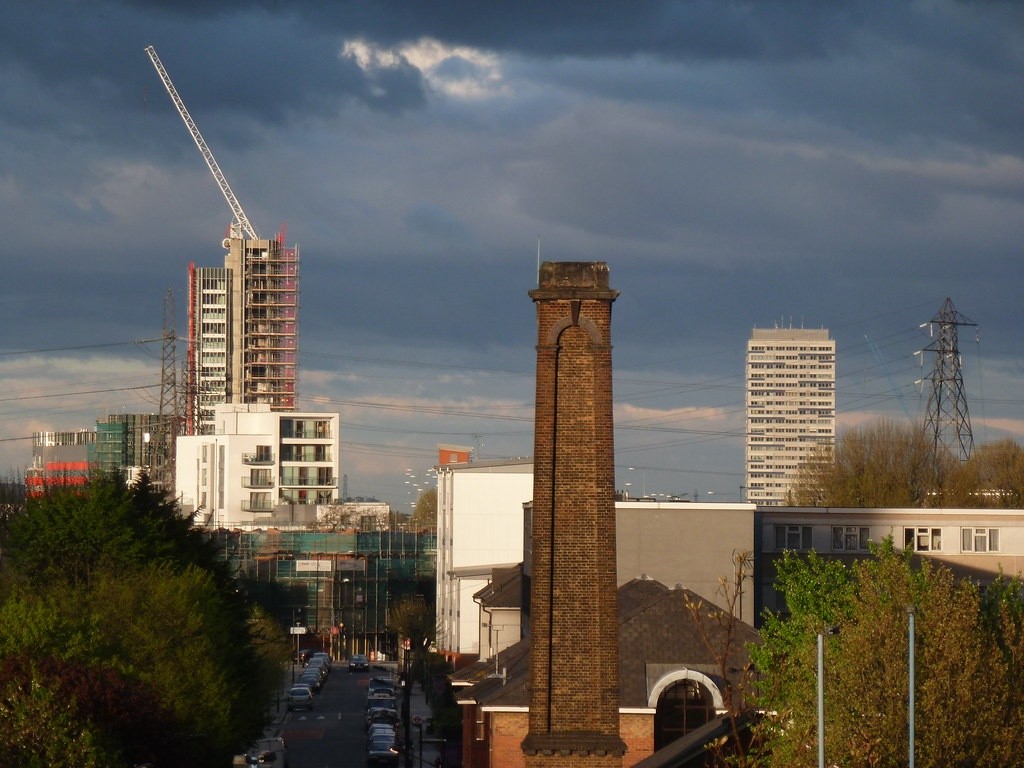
[144,43,259,240]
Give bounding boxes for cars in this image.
[348,654,369,672]
[287,652,331,713]
[368,678,398,762]
